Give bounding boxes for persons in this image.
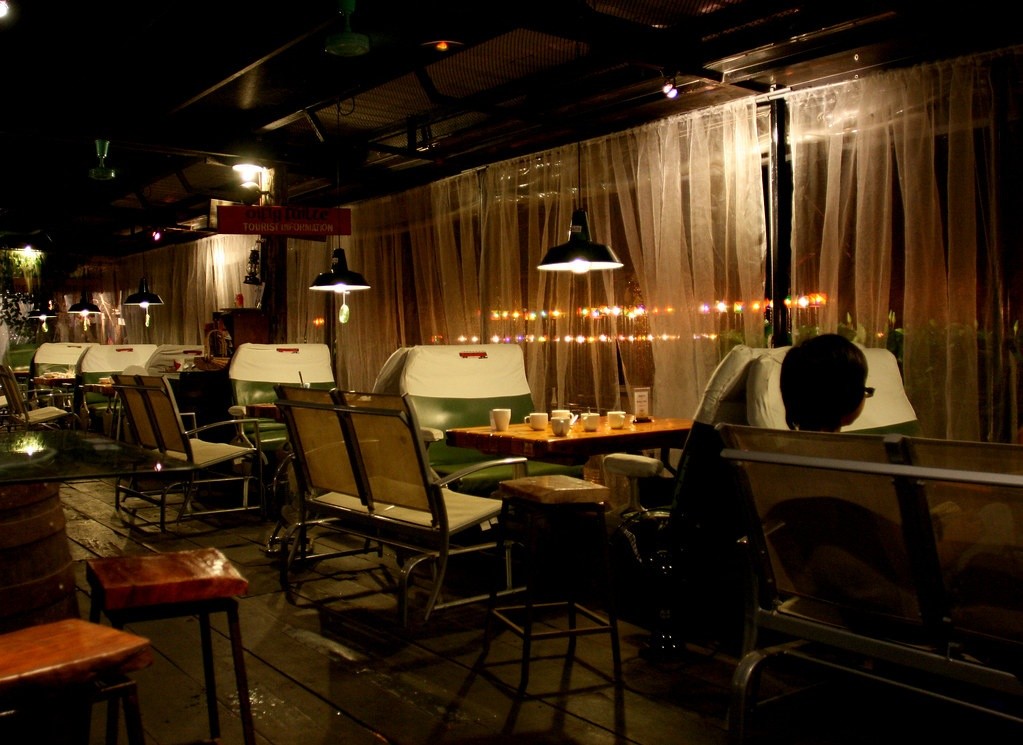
[779,333,1023,643]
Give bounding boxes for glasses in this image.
[864,387,876,398]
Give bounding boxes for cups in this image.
[45,370,50,373]
[581,413,599,430]
[524,412,548,430]
[492,409,511,430]
[551,417,570,435]
[552,410,574,422]
[608,412,626,427]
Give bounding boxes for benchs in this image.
[0,364,68,432]
[368,345,585,494]
[273,383,526,632]
[229,342,338,451]
[111,374,261,535]
[609,344,925,659]
[3,342,205,416]
[716,422,1022,745]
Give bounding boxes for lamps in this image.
[536,140,625,275]
[660,66,681,99]
[124,251,164,306]
[68,268,101,314]
[28,305,56,319]
[309,206,371,293]
[243,234,260,286]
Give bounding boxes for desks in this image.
[445,412,693,480]
[34,376,77,387]
[247,402,284,422]
[0,429,198,634]
[12,370,30,377]
[79,384,116,396]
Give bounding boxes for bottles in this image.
[236,293,244,307]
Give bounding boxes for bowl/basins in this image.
[99,378,109,383]
[44,372,58,378]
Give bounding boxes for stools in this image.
[0,620,153,745]
[485,475,622,682]
[86,548,256,745]
